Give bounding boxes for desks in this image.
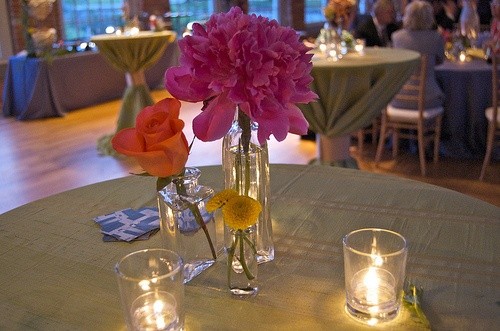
[3,37,179,121]
[91,30,177,154]
[434,63,491,160]
[0,164,500,331]
[298,46,418,168]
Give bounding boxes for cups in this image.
[113,248,185,331]
[343,228,407,327]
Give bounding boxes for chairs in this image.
[480,52,500,180]
[373,55,445,177]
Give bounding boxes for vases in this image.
[157,168,216,285]
[222,104,275,265]
[226,220,258,294]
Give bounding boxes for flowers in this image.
[165,6,320,253]
[208,190,261,288]
[97,97,216,260]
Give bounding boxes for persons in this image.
[321,0,500,160]
